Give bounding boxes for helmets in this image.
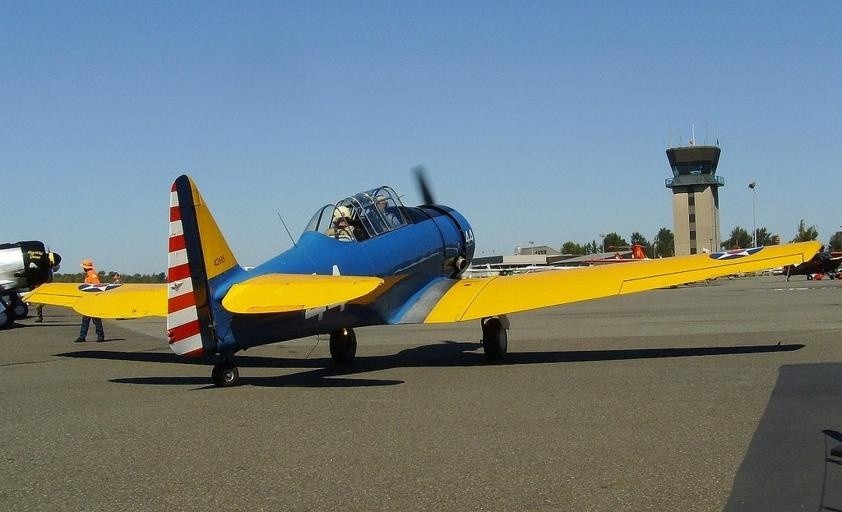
[333,206,351,224]
[375,194,389,205]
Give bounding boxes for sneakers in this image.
[96,335,105,342]
[74,338,86,343]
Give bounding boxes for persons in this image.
[112,271,121,283]
[370,194,402,231]
[322,205,365,244]
[73,258,106,343]
[31,284,45,322]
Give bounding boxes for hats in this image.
[79,259,93,270]
